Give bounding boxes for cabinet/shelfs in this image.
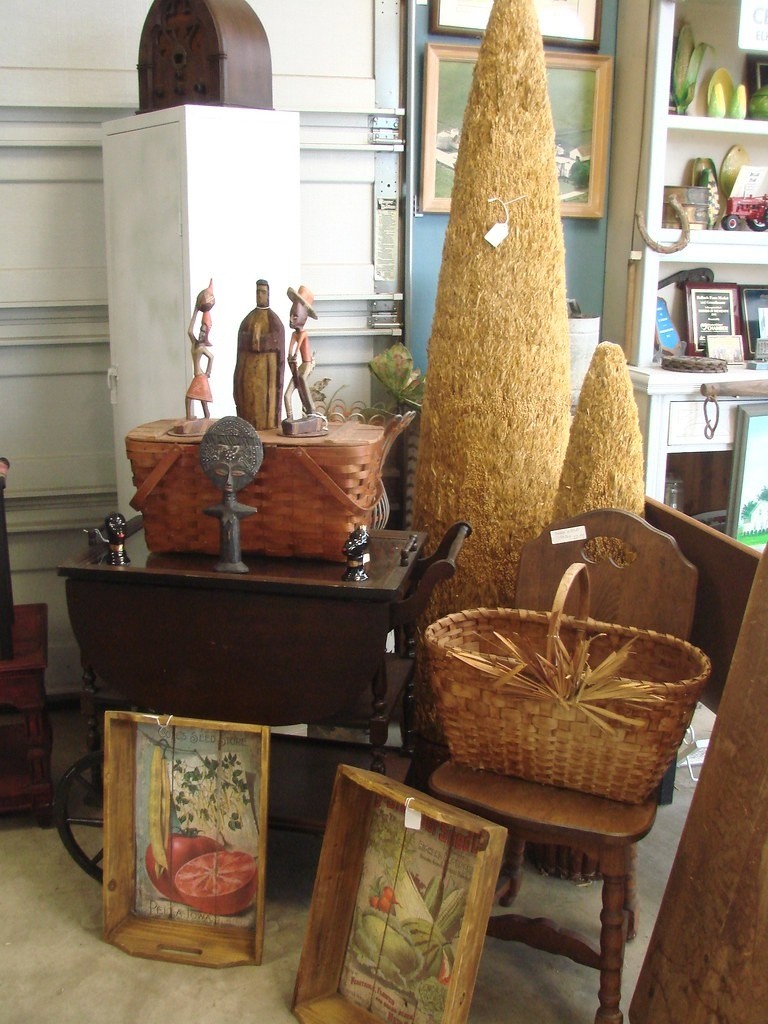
[0,601,55,833]
[54,522,471,881]
[630,2,768,504]
[99,102,307,523]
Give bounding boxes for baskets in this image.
[424,562,712,807]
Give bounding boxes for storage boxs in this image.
[102,708,272,971]
[421,561,712,807]
[125,418,387,567]
[292,762,507,1024]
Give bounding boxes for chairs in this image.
[428,511,699,1024]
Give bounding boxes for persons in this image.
[181,275,332,430]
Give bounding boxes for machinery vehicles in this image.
[720,194,768,232]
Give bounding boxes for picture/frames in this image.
[418,41,615,219]
[430,0,602,51]
